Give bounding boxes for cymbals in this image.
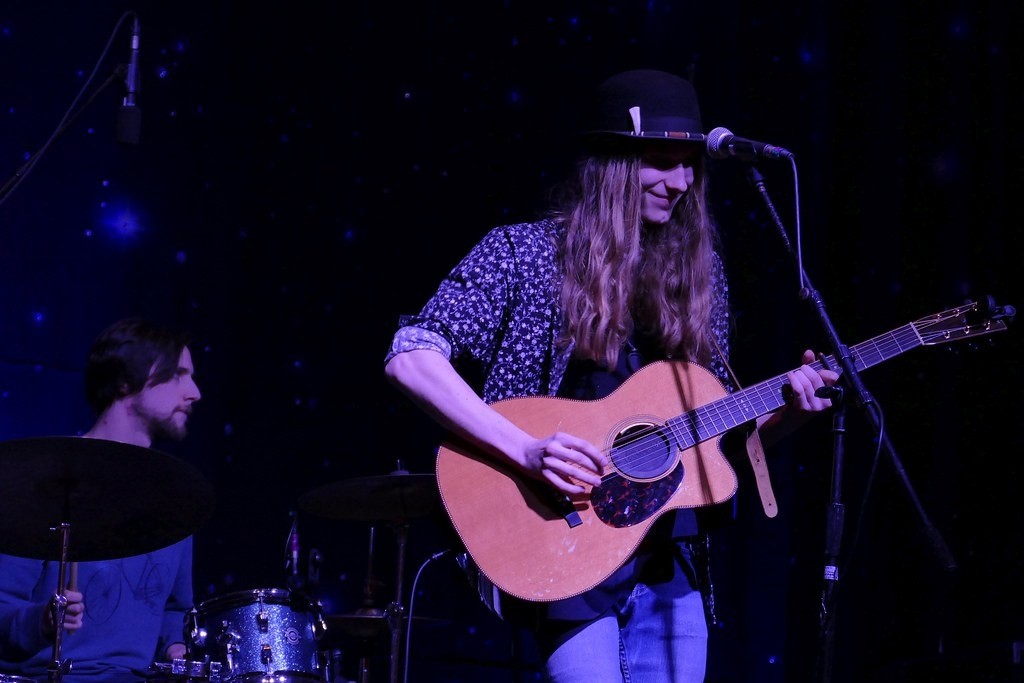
[367,466,438,490]
[337,606,424,621]
[0,434,209,564]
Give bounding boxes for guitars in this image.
[437,292,1018,621]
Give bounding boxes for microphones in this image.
[707,127,794,160]
[124,19,140,106]
[291,525,298,576]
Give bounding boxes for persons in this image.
[381,76,841,683]
[0,316,202,683]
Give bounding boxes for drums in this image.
[181,585,332,683]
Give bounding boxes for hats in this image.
[572,69,706,155]
